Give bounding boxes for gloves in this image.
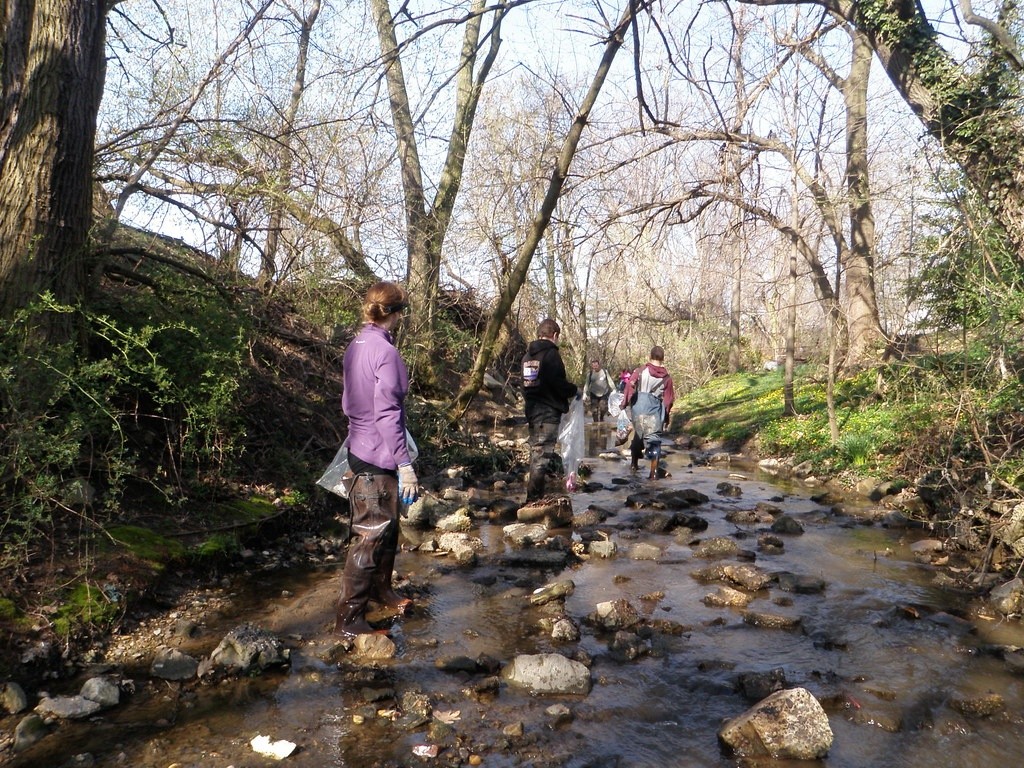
[397,463,419,505]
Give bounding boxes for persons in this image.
[521,318,583,504]
[619,346,675,481]
[582,359,617,423]
[332,281,419,638]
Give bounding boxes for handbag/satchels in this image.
[316,427,419,499]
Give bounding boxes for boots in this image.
[628,457,639,471]
[648,454,658,482]
[334,472,398,642]
[369,513,414,611]
